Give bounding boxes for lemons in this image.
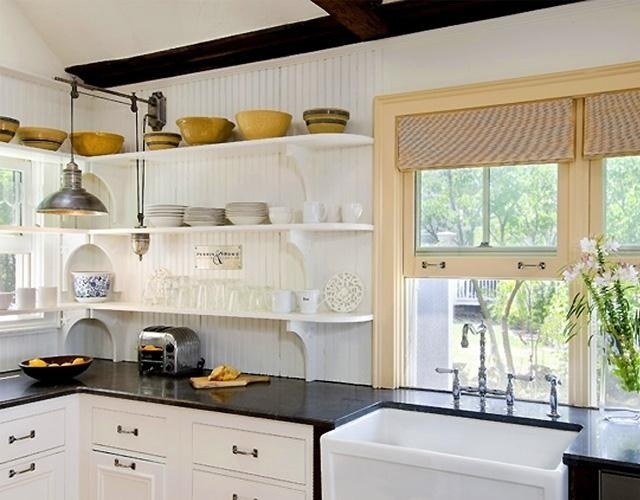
[28,359,46,366]
[72,358,84,365]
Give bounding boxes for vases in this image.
[69,267,114,305]
[597,332,640,426]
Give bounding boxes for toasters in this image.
[136,325,205,378]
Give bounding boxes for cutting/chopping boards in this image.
[189,375,270,390]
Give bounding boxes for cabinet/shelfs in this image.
[183,405,322,500]
[80,393,182,498]
[1,392,80,498]
[569,464,640,500]
[1,130,377,324]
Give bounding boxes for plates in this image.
[144,199,268,227]
[323,270,364,313]
[74,295,109,303]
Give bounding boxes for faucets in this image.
[461,320,487,390]
[544,373,564,418]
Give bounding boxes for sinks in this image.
[318,406,581,500]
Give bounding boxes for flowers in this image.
[552,231,640,394]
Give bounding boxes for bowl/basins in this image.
[142,131,182,151]
[0,115,21,142]
[232,110,292,141]
[175,116,236,146]
[15,126,69,151]
[71,270,111,297]
[19,354,95,382]
[303,108,350,134]
[1,293,14,310]
[67,131,126,156]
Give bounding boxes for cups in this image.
[143,269,328,317]
[266,202,364,225]
[15,286,58,312]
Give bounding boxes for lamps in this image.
[35,77,169,265]
